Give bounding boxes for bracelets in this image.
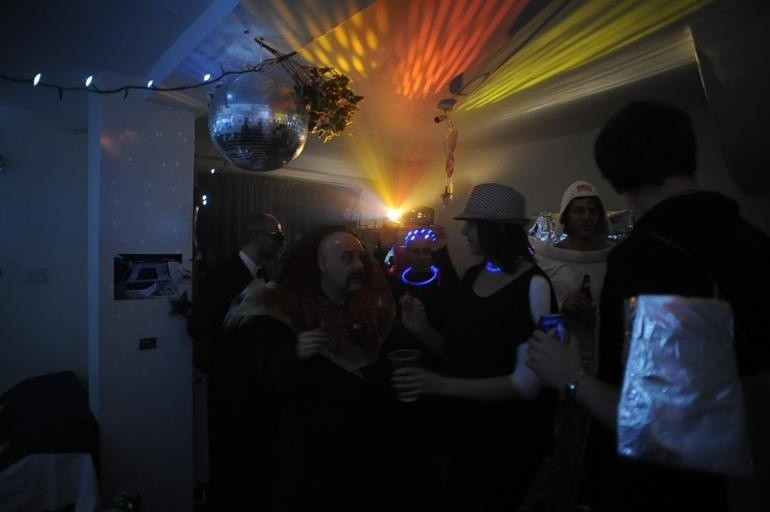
[565,372,587,402]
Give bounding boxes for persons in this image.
[388,184,559,421]
[390,231,446,324]
[257,233,407,394]
[187,211,299,356]
[535,181,616,375]
[526,102,768,510]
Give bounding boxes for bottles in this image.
[580,274,594,298]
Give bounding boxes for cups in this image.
[390,349,422,403]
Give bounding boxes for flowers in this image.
[252,37,364,147]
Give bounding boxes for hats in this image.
[558,181,609,232]
[452,182,535,225]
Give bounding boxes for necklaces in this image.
[486,258,502,273]
[400,265,438,288]
